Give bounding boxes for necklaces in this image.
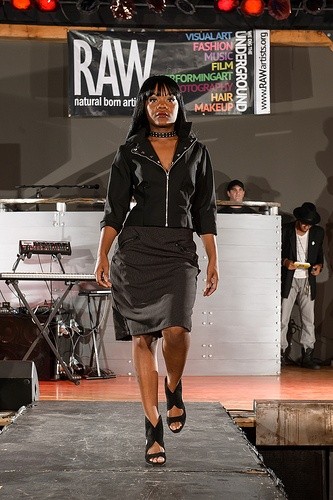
[150,131,177,137]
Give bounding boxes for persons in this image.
[94,75,219,466]
[217,180,258,214]
[281,202,324,369]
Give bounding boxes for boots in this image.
[302,346,320,369]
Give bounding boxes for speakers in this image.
[0,312,79,381]
[0,359,39,411]
[258,449,328,500]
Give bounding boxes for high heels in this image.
[165,376,186,434]
[145,415,167,467]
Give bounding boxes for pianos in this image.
[0,272,96,385]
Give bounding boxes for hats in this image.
[293,202,321,224]
